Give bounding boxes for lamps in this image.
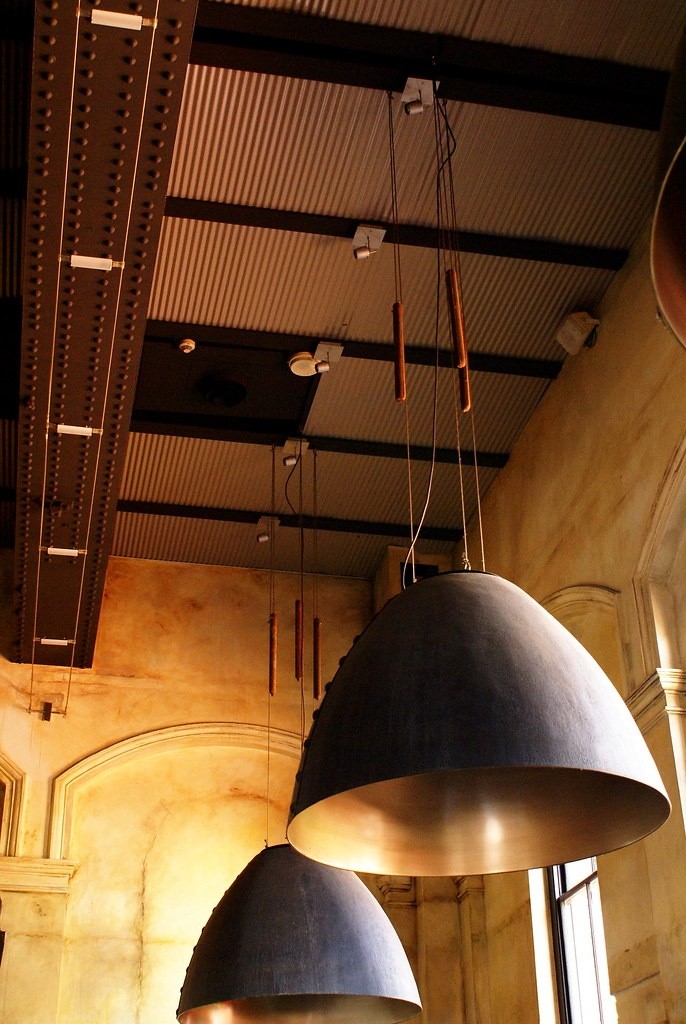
[286,52,672,878]
[555,312,600,355]
[315,353,330,374]
[353,236,376,260]
[176,352,423,1023]
[257,533,269,543]
[284,446,296,467]
[405,90,423,115]
